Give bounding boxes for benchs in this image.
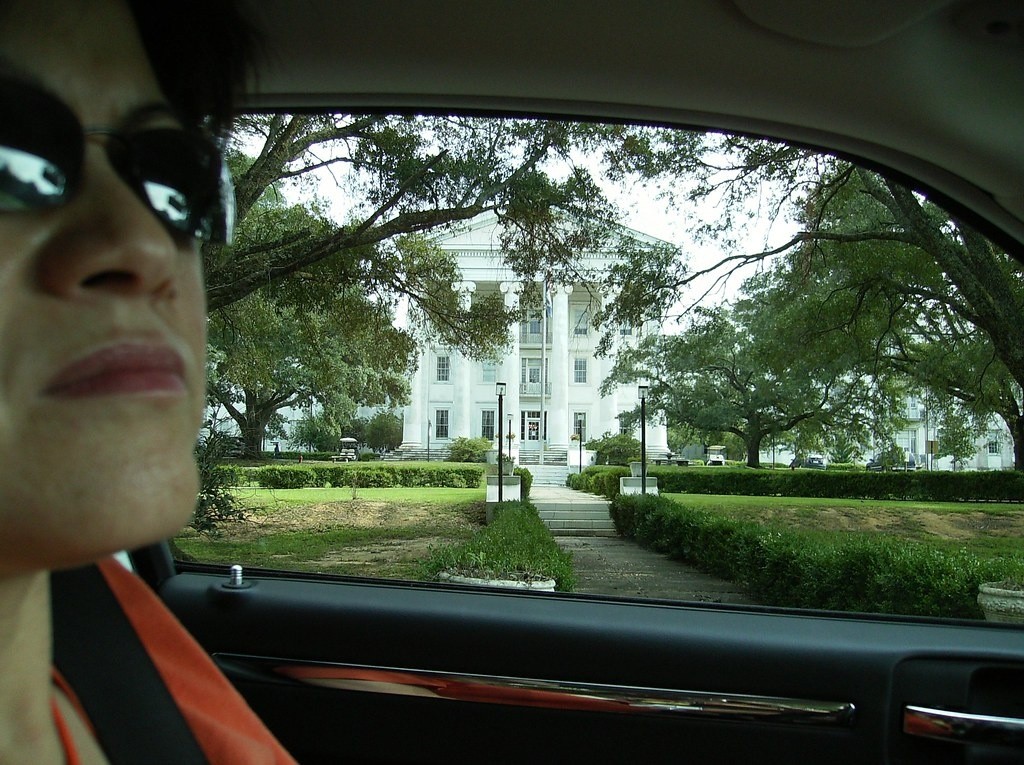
[331,449,356,462]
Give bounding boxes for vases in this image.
[507,438,514,444]
[497,461,514,475]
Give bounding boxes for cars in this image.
[223,437,245,458]
[789,453,827,471]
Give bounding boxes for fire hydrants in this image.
[299,455,303,463]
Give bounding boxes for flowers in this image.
[495,453,516,462]
[506,432,516,438]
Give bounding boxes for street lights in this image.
[578,412,584,473]
[507,413,514,461]
[494,382,506,496]
[637,385,649,495]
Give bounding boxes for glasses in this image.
[0,64,236,248]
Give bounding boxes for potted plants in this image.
[977,561,1024,624]
[627,456,649,477]
[438,550,557,593]
[571,435,578,445]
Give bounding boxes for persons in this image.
[792,460,795,471]
[2,0,253,764]
[275,444,279,459]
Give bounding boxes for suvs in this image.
[865,449,917,471]
